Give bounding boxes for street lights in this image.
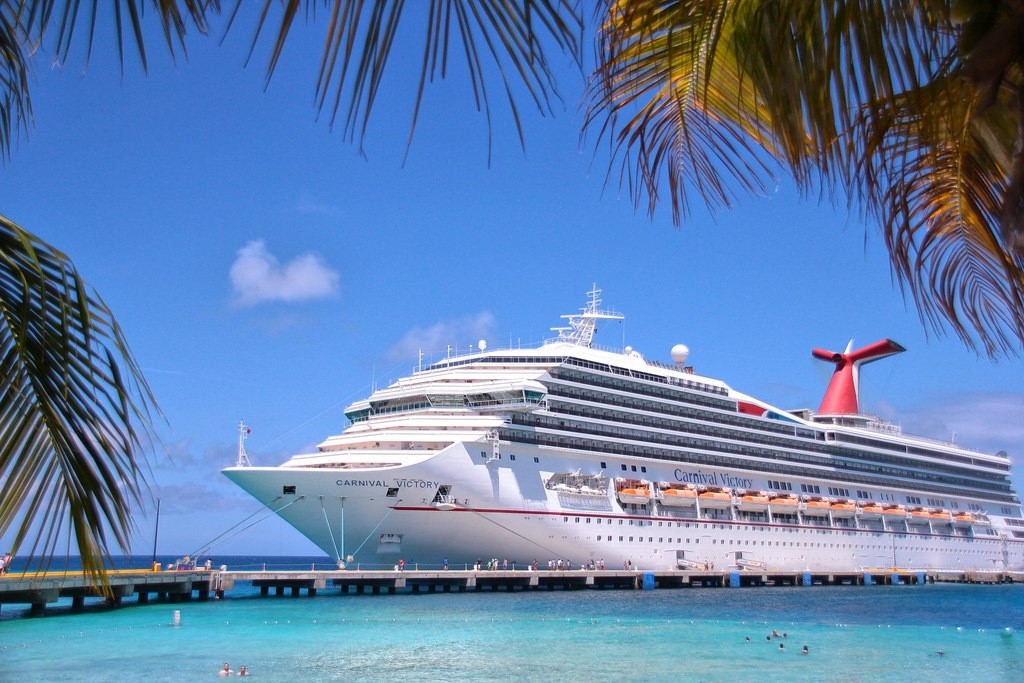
[152,497,161,570]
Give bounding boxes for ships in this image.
[222,282,1023,571]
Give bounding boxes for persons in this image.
[399,559,404,571]
[487,559,499,570]
[705,560,714,570]
[0,553,12,575]
[802,646,809,653]
[623,560,631,570]
[586,558,605,570]
[533,559,537,570]
[219,663,233,676]
[443,557,448,570]
[237,665,249,675]
[779,643,784,650]
[766,637,770,640]
[548,559,570,571]
[475,558,482,570]
[746,636,750,642]
[772,630,787,638]
[503,558,508,569]
[206,559,212,570]
